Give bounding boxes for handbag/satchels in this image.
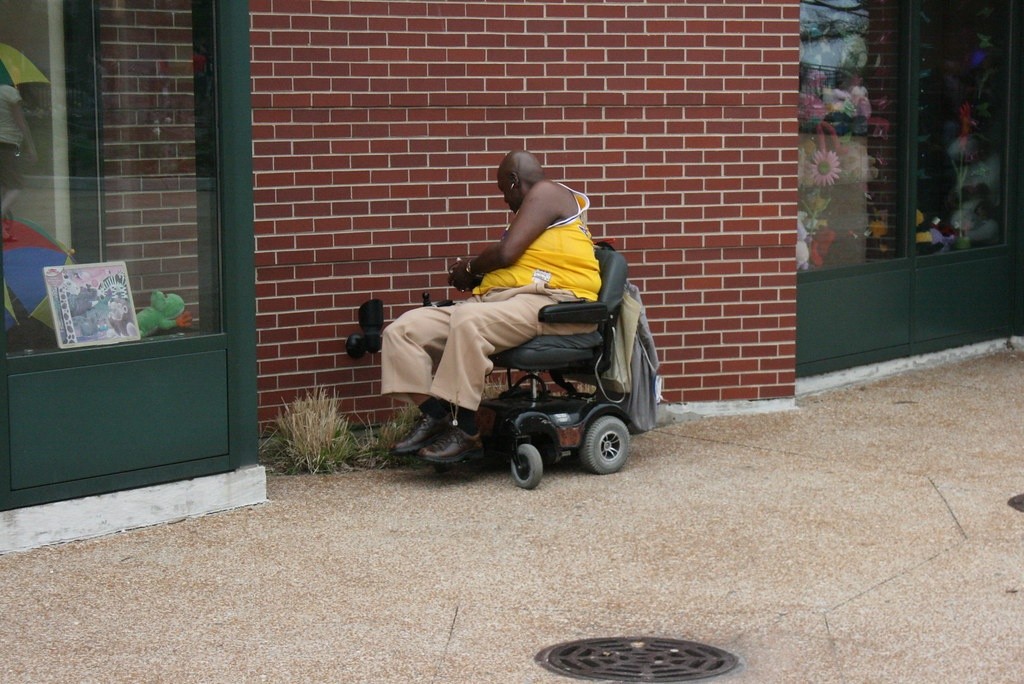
[571,292,641,394]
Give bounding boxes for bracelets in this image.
[466,259,485,278]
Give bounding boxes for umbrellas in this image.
[0,215,80,333]
[0,43,52,90]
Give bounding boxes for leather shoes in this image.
[416,421,485,464]
[391,412,450,456]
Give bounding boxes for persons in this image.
[1,84,39,244]
[382,149,603,464]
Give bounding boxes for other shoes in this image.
[2,232,17,243]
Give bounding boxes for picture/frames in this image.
[41,259,142,349]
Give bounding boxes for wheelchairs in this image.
[416,240,640,490]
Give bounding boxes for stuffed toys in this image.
[135,290,193,338]
